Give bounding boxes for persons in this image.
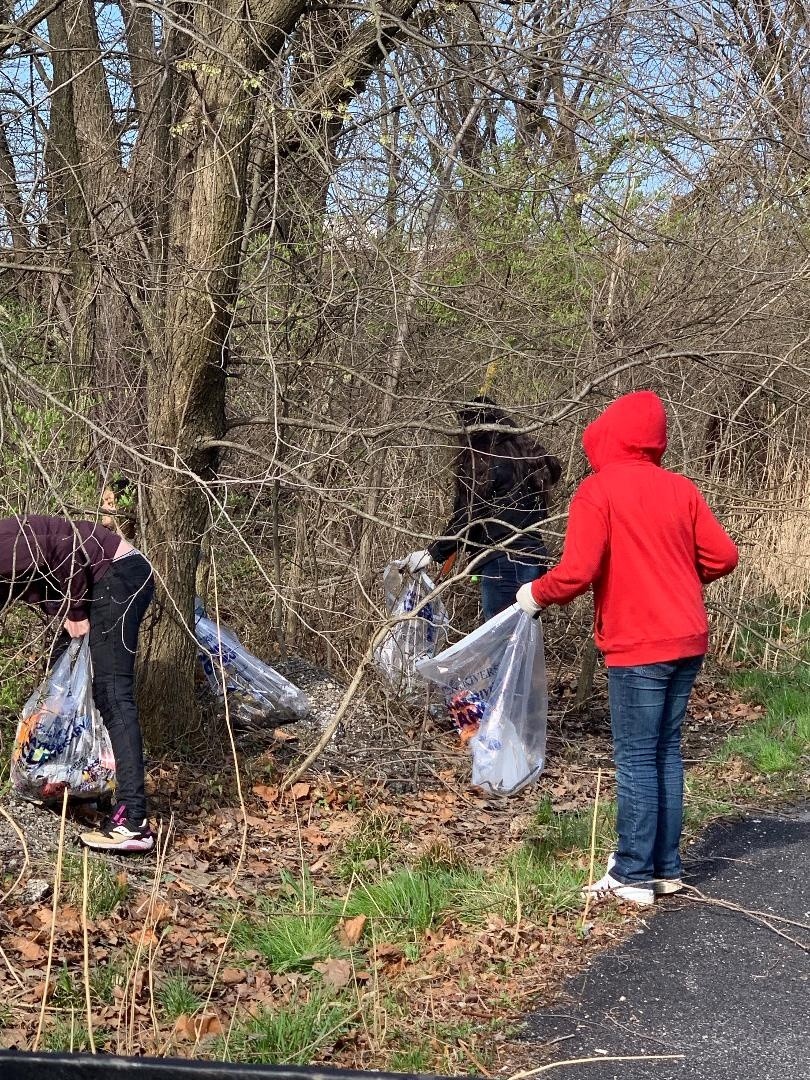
[516,390,738,908]
[405,396,561,622]
[0,517,155,849]
[705,395,729,476]
[101,479,138,541]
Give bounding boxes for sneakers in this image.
[580,874,654,905]
[82,818,154,850]
[607,850,684,893]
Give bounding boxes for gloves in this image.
[398,548,434,573]
[515,581,544,621]
